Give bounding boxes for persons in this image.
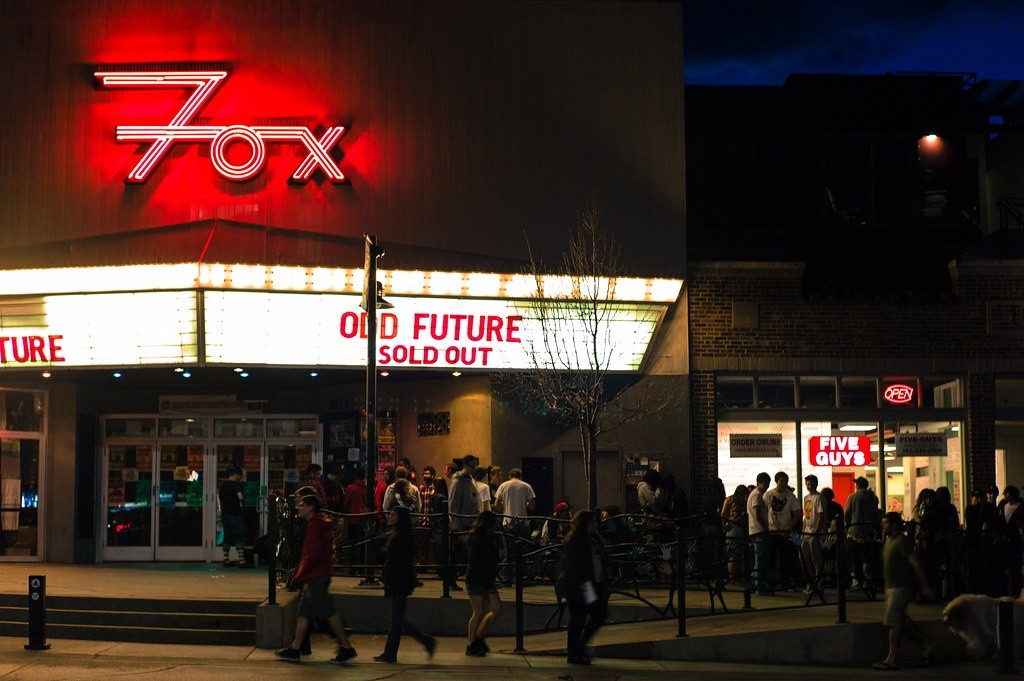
[638,469,1024,670]
[218,455,631,665]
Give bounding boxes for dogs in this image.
[942,588,1024,664]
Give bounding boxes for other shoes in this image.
[495,583,504,589]
[223,562,238,567]
[466,637,490,657]
[567,654,591,665]
[372,653,397,663]
[239,563,255,569]
[426,638,436,658]
[449,584,464,591]
[847,584,860,592]
[416,581,424,587]
[803,589,823,596]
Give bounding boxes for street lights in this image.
[357,233,389,586]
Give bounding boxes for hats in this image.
[986,485,998,496]
[853,477,868,488]
[555,501,568,511]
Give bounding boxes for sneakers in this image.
[330,646,358,664]
[274,646,300,661]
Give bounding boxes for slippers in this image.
[919,637,939,666]
[872,662,897,670]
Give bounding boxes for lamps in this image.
[450,371,462,377]
[232,368,250,379]
[308,369,320,378]
[42,370,51,378]
[173,368,192,379]
[112,370,123,378]
[380,370,390,377]
